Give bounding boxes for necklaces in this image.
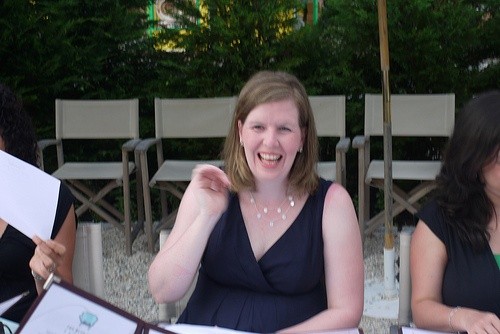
[246,186,296,229]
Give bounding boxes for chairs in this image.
[352,92,457,253]
[33,98,145,257]
[135,93,238,253]
[308,94,351,190]
[69,220,105,299]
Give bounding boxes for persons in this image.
[146,68,366,334]
[0,86,78,334]
[407,91,500,334]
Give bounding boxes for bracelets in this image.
[447,305,460,330]
[30,264,53,282]
